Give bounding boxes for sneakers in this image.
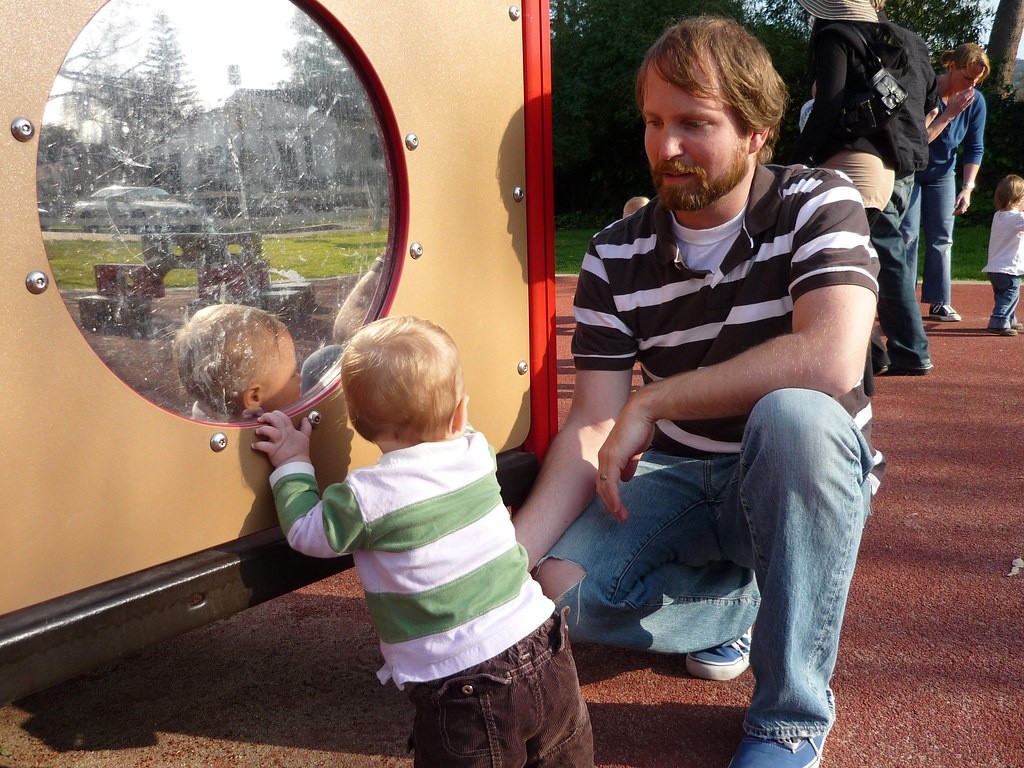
[929,303,962,322]
[727,734,829,768]
[685,626,751,681]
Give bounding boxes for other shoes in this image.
[874,364,888,377]
[987,327,1018,336]
[886,357,934,375]
[1011,323,1023,330]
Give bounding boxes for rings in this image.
[600,474,607,480]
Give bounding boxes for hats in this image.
[797,0,879,22]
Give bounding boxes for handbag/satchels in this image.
[833,23,909,139]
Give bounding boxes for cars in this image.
[37,207,51,231]
[72,185,203,235]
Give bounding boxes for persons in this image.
[251,317,594,768]
[875,1,935,373]
[300,251,386,395]
[514,17,891,768]
[796,0,903,397]
[900,43,991,320]
[982,174,1024,336]
[624,197,649,219]
[174,304,303,423]
